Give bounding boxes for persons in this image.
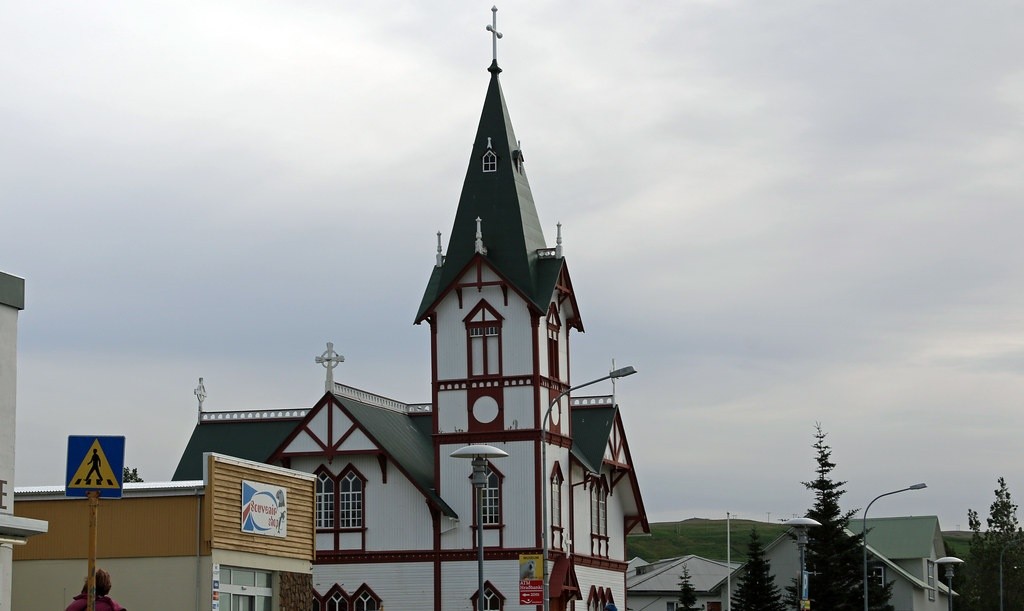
[63,567,127,611]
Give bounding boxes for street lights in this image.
[864,483,928,611]
[934,556,965,611]
[541,365,638,611]
[449,445,509,611]
[781,518,823,611]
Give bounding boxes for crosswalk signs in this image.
[65,435,126,499]
